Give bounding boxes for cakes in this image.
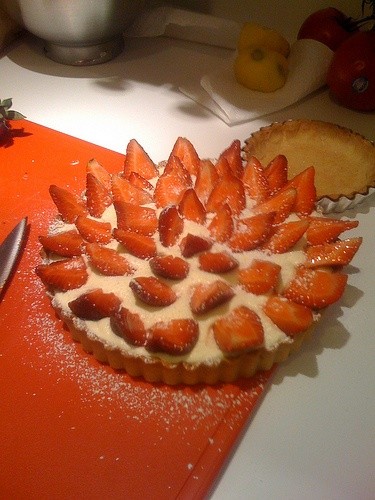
[34,135,362,388]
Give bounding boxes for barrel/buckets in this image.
[5,0,147,65]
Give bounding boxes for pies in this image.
[245,119,375,203]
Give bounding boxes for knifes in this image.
[0,216,28,293]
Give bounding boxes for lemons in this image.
[235,20,293,91]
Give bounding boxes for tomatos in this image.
[296,7,374,110]
